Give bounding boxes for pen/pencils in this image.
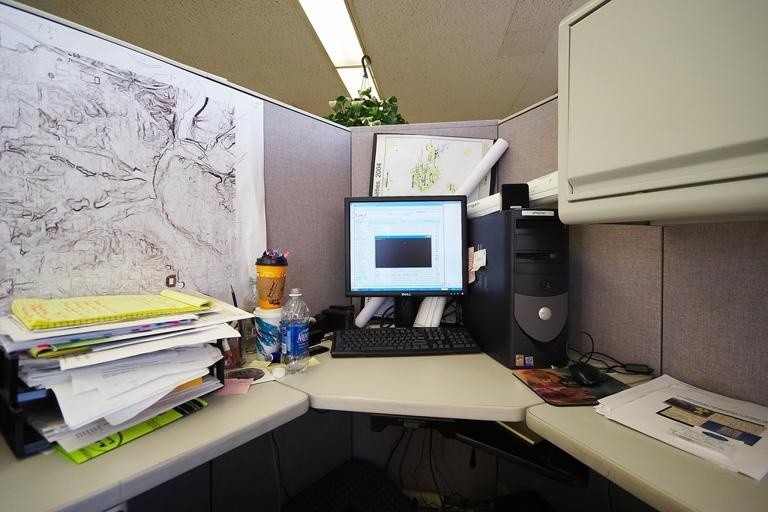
[231,285,244,337]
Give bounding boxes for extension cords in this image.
[401,488,445,509]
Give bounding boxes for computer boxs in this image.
[462,209,570,370]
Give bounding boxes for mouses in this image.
[568,363,601,387]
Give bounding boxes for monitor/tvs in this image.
[344,195,469,328]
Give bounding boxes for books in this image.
[0,288,256,465]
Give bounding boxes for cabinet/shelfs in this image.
[556,0,767,225]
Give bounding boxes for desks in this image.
[244,336,543,427]
[526,374,767,512]
[1,373,309,512]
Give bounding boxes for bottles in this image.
[282,288,310,374]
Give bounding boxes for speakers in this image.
[501,183,530,211]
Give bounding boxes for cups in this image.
[255,257,288,309]
[253,307,285,364]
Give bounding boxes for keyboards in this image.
[330,327,481,358]
